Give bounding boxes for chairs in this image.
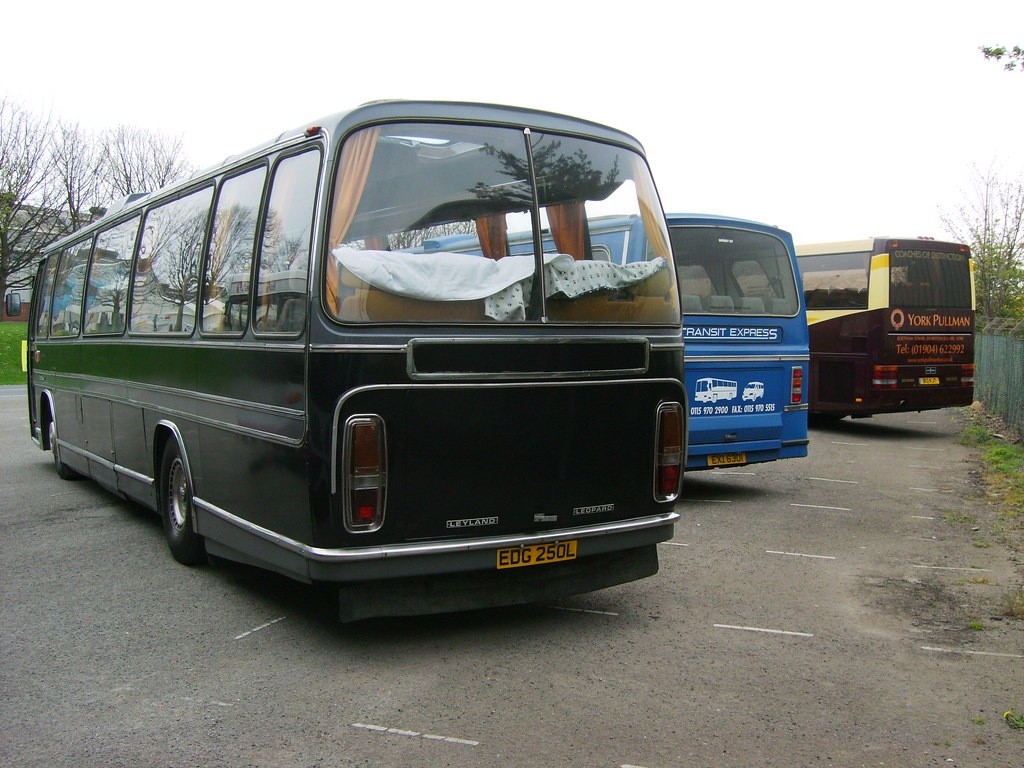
[708,295,734,313]
[804,281,932,307]
[683,294,704,312]
[768,299,794,314]
[740,297,765,314]
[276,298,305,332]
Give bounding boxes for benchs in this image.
[335,253,672,318]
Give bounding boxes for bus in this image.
[4,100,690,624]
[382,211,808,472]
[680,239,979,421]
